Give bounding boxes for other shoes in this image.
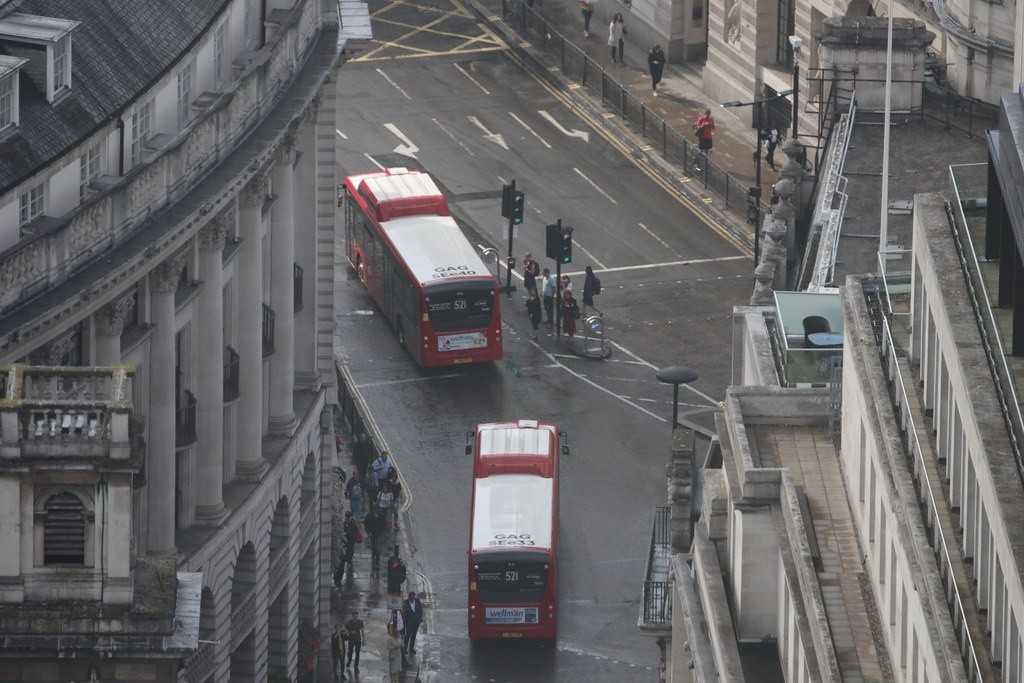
[346,661,359,672]
[543,319,553,325]
[584,30,591,38]
[612,57,625,66]
[390,522,400,530]
[405,648,417,654]
[695,165,701,172]
[653,90,657,97]
[765,157,776,174]
[566,338,577,345]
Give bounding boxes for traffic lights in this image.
[388,558,400,593]
[560,228,572,264]
[512,192,524,225]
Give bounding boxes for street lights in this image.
[656,366,698,435]
[721,89,801,286]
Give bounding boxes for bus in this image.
[336,166,501,368]
[465,420,570,646]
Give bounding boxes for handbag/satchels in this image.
[569,299,581,320]
[693,124,704,136]
[551,291,561,307]
[355,531,362,544]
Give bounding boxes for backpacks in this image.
[592,278,600,295]
[349,483,362,499]
[531,260,540,276]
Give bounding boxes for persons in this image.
[524,288,541,339]
[764,119,781,171]
[647,44,665,97]
[693,108,715,171]
[540,268,556,326]
[560,275,576,344]
[522,253,537,296]
[608,13,626,66]
[330,625,350,680]
[581,265,604,319]
[579,0,593,38]
[345,612,366,672]
[335,432,402,589]
[402,592,423,654]
[387,628,406,683]
